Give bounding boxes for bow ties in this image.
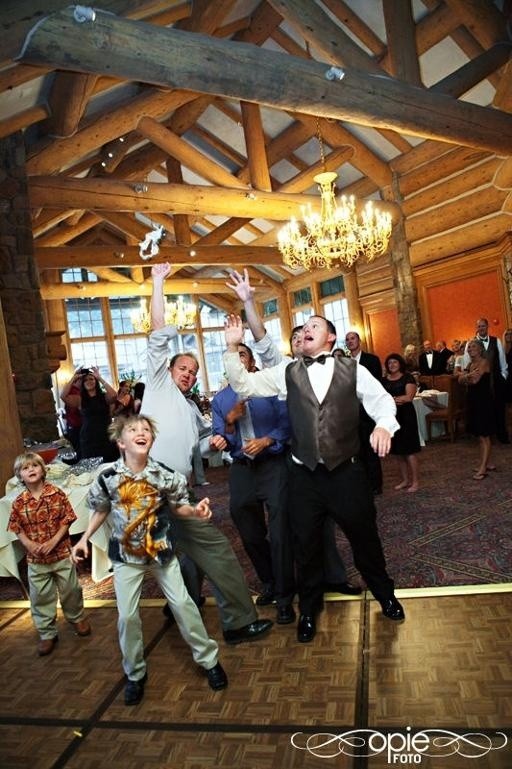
[425,351,432,354]
[303,354,326,366]
[480,339,488,342]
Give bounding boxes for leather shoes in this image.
[256,584,275,606]
[124,671,147,704]
[379,595,405,619]
[206,660,228,690]
[37,635,59,656]
[222,619,273,644]
[275,604,296,625]
[163,594,206,617]
[297,614,317,643]
[336,583,363,594]
[72,619,90,635]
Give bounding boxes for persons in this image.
[60,363,213,488]
[344,332,383,382]
[225,268,362,624]
[211,342,297,625]
[139,261,275,645]
[223,312,404,643]
[383,353,422,493]
[332,348,344,357]
[6,451,91,657]
[402,317,512,480]
[69,413,229,706]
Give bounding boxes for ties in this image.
[235,397,256,460]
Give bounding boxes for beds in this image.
[411,392,455,447]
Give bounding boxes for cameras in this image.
[81,369,89,374]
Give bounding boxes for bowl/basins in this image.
[36,448,58,465]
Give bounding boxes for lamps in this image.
[132,296,197,336]
[277,116,394,272]
[325,66,345,81]
[73,4,96,23]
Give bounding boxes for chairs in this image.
[29,448,59,464]
[419,375,433,392]
[425,374,465,445]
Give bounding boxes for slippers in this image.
[474,473,489,480]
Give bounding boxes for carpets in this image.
[0,443,511,605]
